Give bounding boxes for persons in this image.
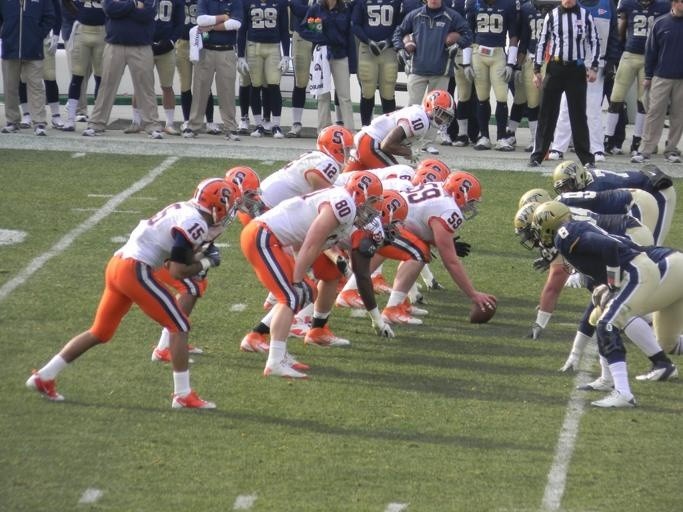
[470,0,522,150]
[516,2,552,151]
[515,205,656,371]
[236,125,358,310]
[122,0,185,137]
[182,0,246,142]
[554,160,679,248]
[509,2,525,142]
[340,89,456,174]
[526,203,661,407]
[228,1,264,132]
[63,0,107,131]
[532,246,683,390]
[348,0,407,127]
[297,167,445,313]
[287,0,345,134]
[81,1,168,139]
[238,0,290,138]
[330,159,451,309]
[17,0,63,130]
[548,0,620,164]
[0,0,54,135]
[520,184,659,244]
[336,172,499,327]
[239,189,409,355]
[151,166,264,363]
[450,0,482,146]
[27,178,240,410]
[175,1,223,135]
[527,0,597,168]
[603,0,673,158]
[240,170,385,379]
[393,0,474,154]
[629,0,683,165]
[312,0,356,139]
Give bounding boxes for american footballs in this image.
[469,296,497,324]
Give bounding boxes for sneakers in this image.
[52,114,64,129]
[441,133,452,146]
[652,146,658,154]
[189,345,202,354]
[304,323,351,349]
[584,163,595,168]
[164,126,181,135]
[381,303,422,324]
[576,378,615,392]
[525,144,534,152]
[473,137,490,150]
[20,112,31,128]
[505,127,517,147]
[631,153,650,163]
[263,291,277,309]
[420,145,439,155]
[171,390,217,410]
[401,297,428,315]
[611,146,623,155]
[182,121,189,133]
[272,126,284,139]
[287,122,302,137]
[63,120,75,131]
[370,273,392,295]
[335,121,344,126]
[152,348,194,364]
[1,123,20,133]
[494,138,516,152]
[250,125,264,138]
[82,127,105,136]
[665,154,680,163]
[124,122,140,133]
[591,390,635,409]
[76,114,87,122]
[184,128,195,138]
[225,130,240,142]
[452,134,470,147]
[206,123,222,135]
[240,333,270,353]
[64,101,68,112]
[283,353,309,369]
[151,130,163,139]
[33,125,46,136]
[264,361,307,379]
[630,145,638,156]
[635,362,678,382]
[544,150,563,161]
[239,117,249,133]
[427,279,447,291]
[527,155,541,167]
[594,151,606,162]
[604,142,610,152]
[335,289,366,309]
[288,315,311,338]
[264,122,273,136]
[26,370,64,401]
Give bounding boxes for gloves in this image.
[278,57,290,74]
[600,289,616,312]
[591,283,609,307]
[293,282,306,308]
[337,256,352,278]
[204,245,220,267]
[397,49,409,65]
[408,282,422,304]
[533,258,550,274]
[453,236,471,257]
[369,40,381,57]
[445,43,460,58]
[602,60,614,82]
[559,359,579,372]
[47,35,58,56]
[376,324,394,338]
[377,38,390,53]
[524,323,543,341]
[237,57,249,77]
[514,66,523,84]
[500,64,514,83]
[565,273,581,288]
[463,64,474,83]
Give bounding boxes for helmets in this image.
[553,160,585,194]
[417,159,450,179]
[193,177,235,227]
[318,125,360,168]
[225,166,263,214]
[443,171,483,220]
[345,171,383,231]
[424,89,455,132]
[519,189,552,208]
[380,190,408,243]
[530,201,571,249]
[412,168,442,186]
[514,202,540,250]
